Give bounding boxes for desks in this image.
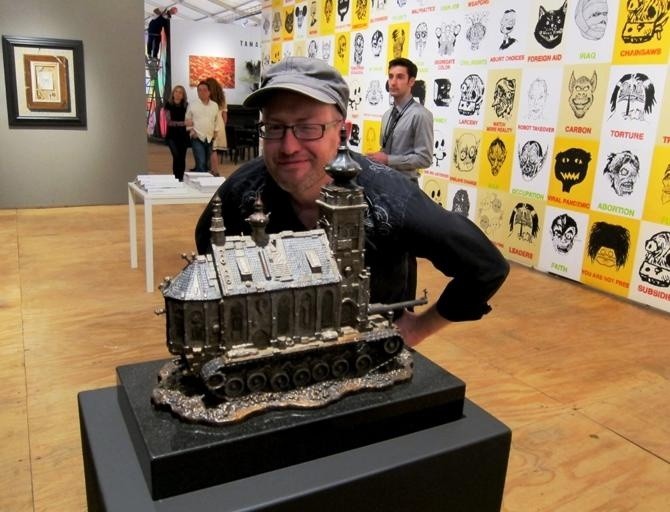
[127,180,228,293]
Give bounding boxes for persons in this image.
[146,5,178,62]
[362,56,437,314]
[162,82,195,182]
[194,54,513,353]
[185,80,221,177]
[202,76,230,178]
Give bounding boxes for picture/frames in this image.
[1,35,88,129]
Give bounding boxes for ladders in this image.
[145,53,161,137]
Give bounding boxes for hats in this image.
[243,56,349,118]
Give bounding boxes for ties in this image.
[385,108,397,154]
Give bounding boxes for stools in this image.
[217,105,260,163]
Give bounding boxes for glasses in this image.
[256,120,342,140]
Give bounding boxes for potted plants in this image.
[240,60,260,91]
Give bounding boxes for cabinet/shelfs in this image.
[77,344,514,512]
